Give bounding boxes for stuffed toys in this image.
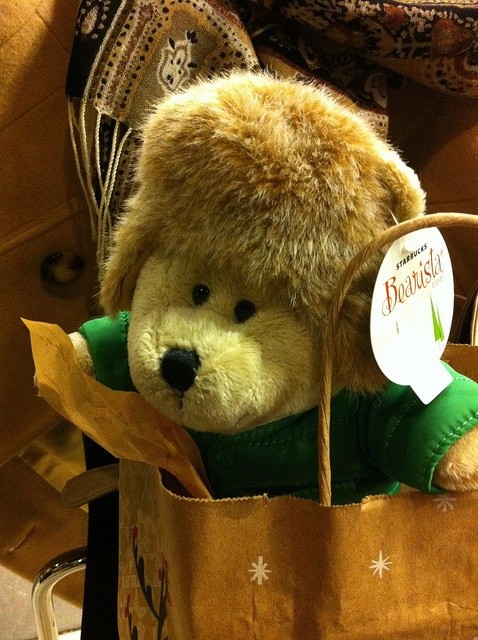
[33,68,477,504]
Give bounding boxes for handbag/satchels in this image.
[117,213,477,639]
